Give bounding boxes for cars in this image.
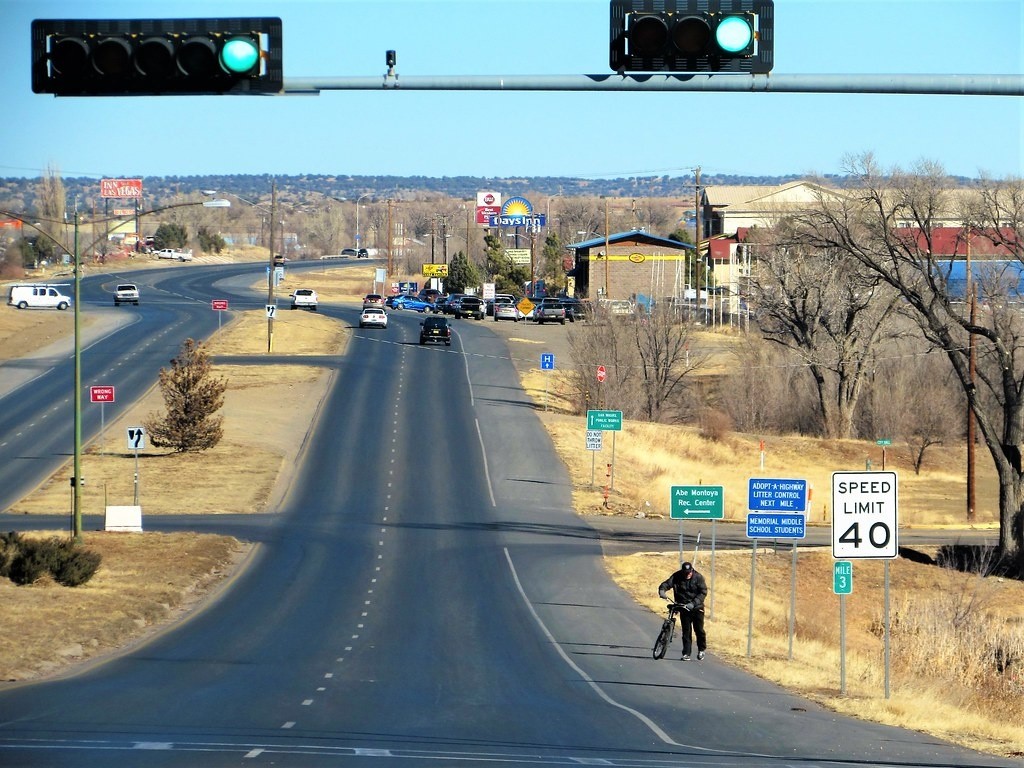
[362,294,386,313]
[113,284,140,307]
[357,248,368,258]
[340,248,357,256]
[288,288,318,311]
[274,255,282,268]
[382,287,487,321]
[359,307,389,328]
[419,316,452,346]
[490,293,636,325]
[684,286,725,304]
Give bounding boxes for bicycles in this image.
[652,596,698,661]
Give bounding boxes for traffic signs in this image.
[587,410,622,431]
[669,485,725,520]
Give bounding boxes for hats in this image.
[682,562,692,574]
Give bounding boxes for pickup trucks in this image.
[150,248,193,263]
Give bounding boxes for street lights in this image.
[445,234,470,289]
[201,189,278,353]
[421,233,448,281]
[506,232,535,297]
[1,197,232,537]
[576,198,611,298]
[356,193,375,258]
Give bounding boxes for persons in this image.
[659,562,708,660]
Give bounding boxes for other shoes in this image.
[697,650,705,660]
[681,654,690,661]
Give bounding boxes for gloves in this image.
[660,590,667,599]
[685,602,694,609]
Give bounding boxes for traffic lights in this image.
[44,28,269,82]
[623,10,760,60]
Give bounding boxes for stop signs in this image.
[597,365,606,382]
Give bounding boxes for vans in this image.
[6,285,71,311]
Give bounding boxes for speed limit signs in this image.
[832,474,899,562]
